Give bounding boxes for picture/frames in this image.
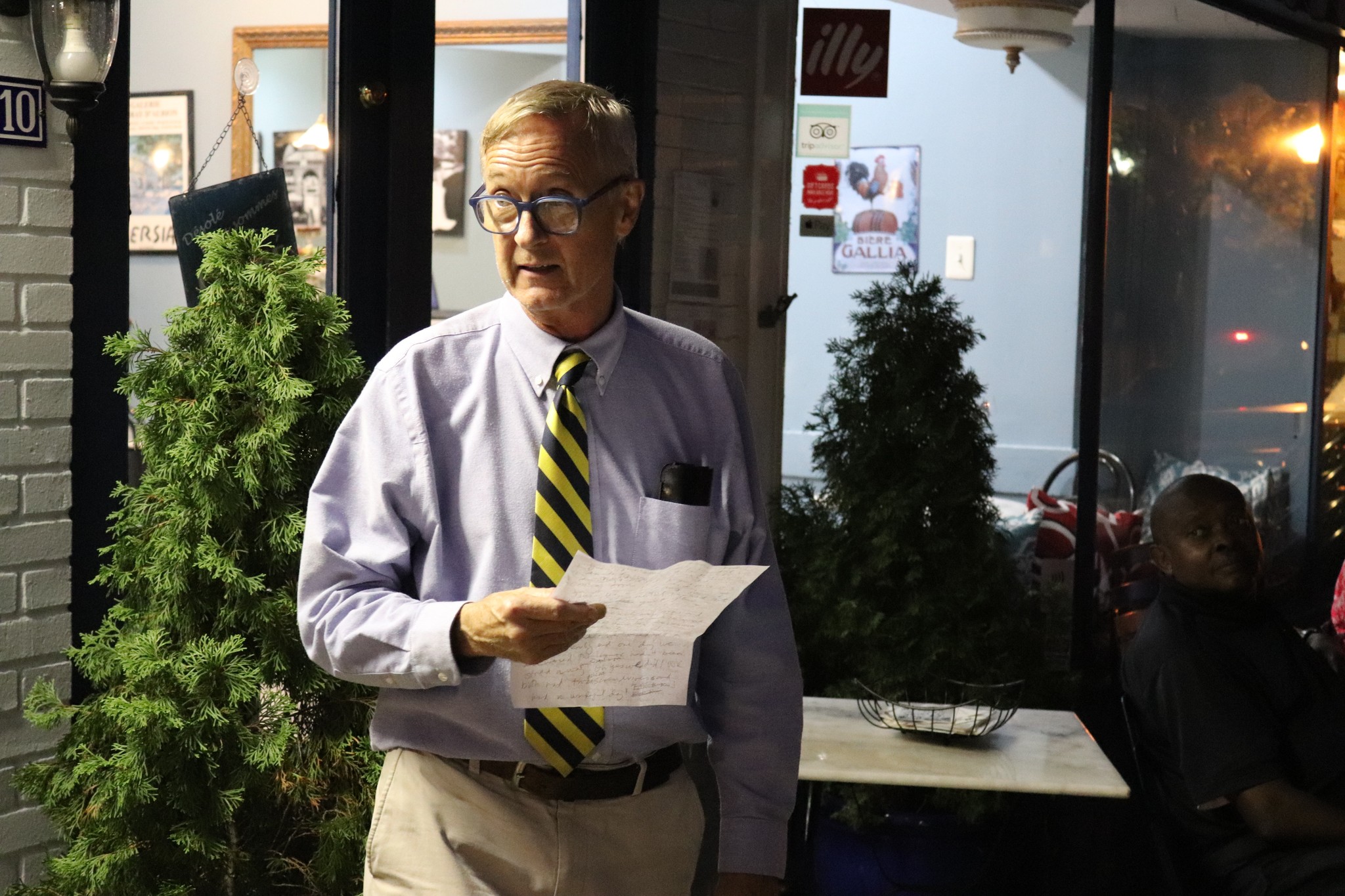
[130,90,195,254]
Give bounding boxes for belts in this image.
[461,741,686,802]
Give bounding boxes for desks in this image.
[800,697,1131,896]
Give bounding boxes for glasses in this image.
[468,174,635,237]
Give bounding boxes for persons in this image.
[1120,474,1345,896]
[297,85,803,896]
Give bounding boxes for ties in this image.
[521,347,605,777]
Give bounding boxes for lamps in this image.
[0,0,120,140]
[952,0,1090,74]
